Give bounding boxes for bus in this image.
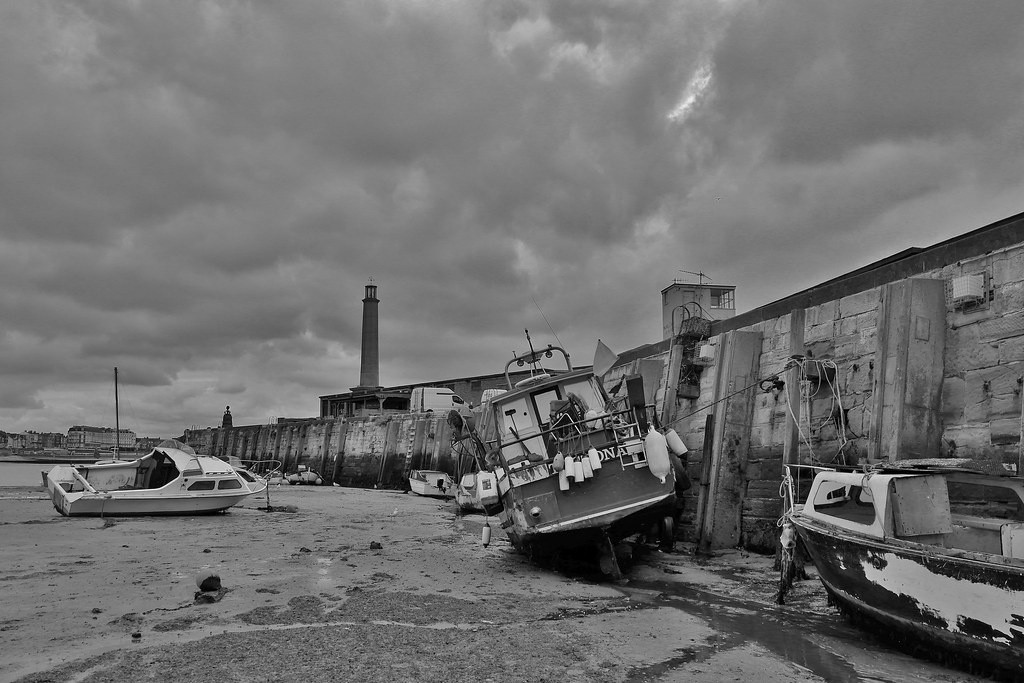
[68,448,95,457]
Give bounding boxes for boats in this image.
[486,327,679,556]
[408,469,458,497]
[265,464,322,486]
[455,419,499,511]
[41,448,282,518]
[782,462,1024,679]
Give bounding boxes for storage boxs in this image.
[554,428,607,454]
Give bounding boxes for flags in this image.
[593,339,619,377]
